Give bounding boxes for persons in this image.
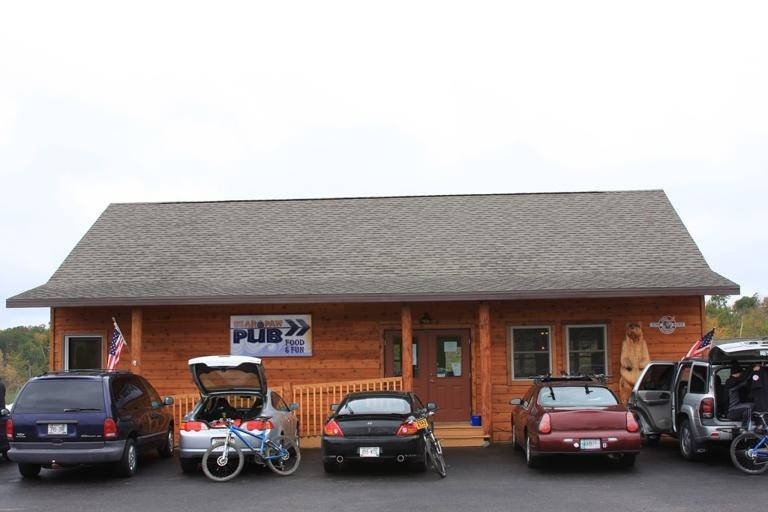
[723,363,768,436]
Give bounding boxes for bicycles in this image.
[730,412,768,475]
[400,403,447,478]
[201,414,300,482]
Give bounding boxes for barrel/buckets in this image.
[471,416,481,426]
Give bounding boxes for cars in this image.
[509,372,641,468]
[179,355,300,472]
[322,392,435,474]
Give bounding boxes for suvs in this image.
[8,368,175,476]
[628,337,768,461]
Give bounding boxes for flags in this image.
[105,323,124,373]
[685,328,715,359]
